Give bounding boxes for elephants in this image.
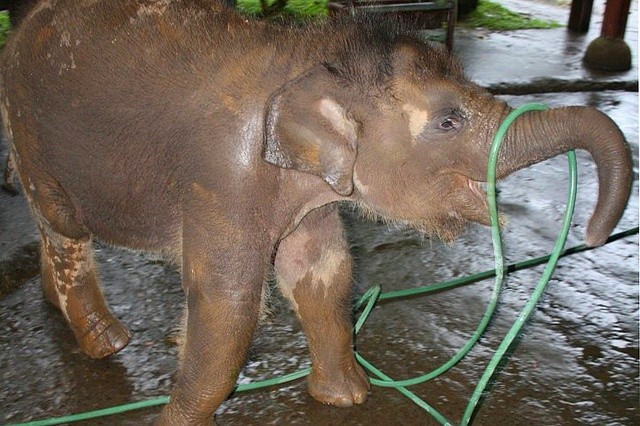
[0,0,633,426]
